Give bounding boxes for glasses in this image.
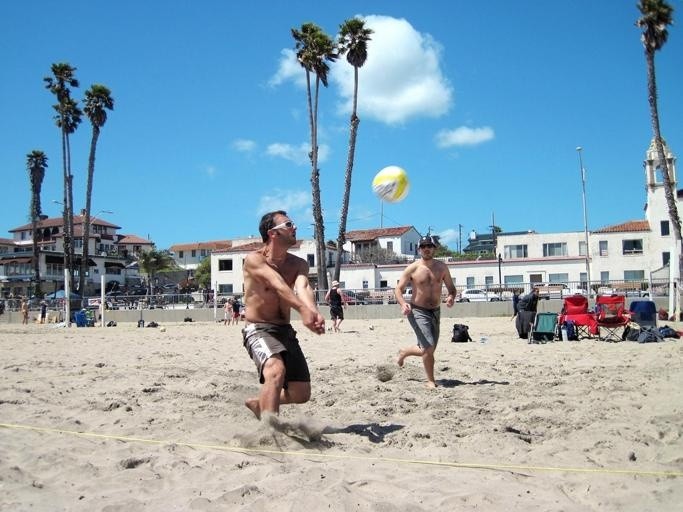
[271,220,293,230]
[421,243,433,249]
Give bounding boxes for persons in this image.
[224,297,240,325]
[0,293,48,325]
[324,280,347,331]
[395,236,456,387]
[314,283,319,301]
[511,288,539,321]
[242,210,324,422]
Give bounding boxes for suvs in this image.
[458,288,505,303]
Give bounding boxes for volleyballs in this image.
[372,166,409,202]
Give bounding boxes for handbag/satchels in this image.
[623,324,680,344]
[452,324,471,342]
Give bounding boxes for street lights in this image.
[575,147,591,315]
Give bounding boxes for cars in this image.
[393,287,412,302]
[105,291,125,302]
[339,290,383,305]
[570,286,649,299]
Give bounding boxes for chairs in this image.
[530,295,656,342]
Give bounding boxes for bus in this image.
[533,282,571,297]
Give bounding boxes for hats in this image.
[416,236,437,249]
[331,280,340,286]
